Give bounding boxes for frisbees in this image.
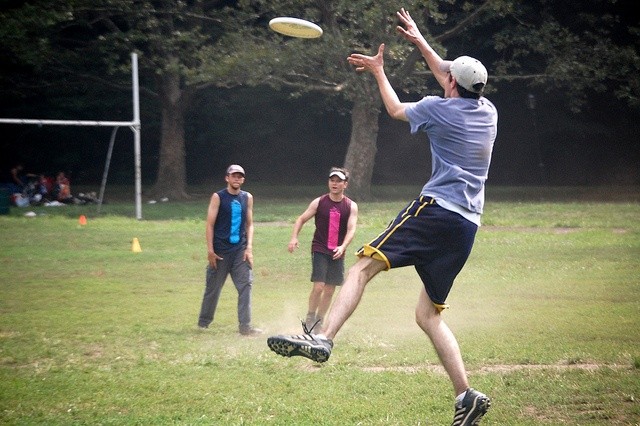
[269,17,323,38]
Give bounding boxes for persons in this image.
[198,164,261,338]
[288,167,359,336]
[267,8,498,426]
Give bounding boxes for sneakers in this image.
[240,325,264,336]
[450,386,492,426]
[268,317,333,363]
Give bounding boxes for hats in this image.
[328,168,349,181]
[438,54,490,94]
[226,163,246,176]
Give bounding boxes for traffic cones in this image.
[131,237,142,253]
[79,214,87,225]
